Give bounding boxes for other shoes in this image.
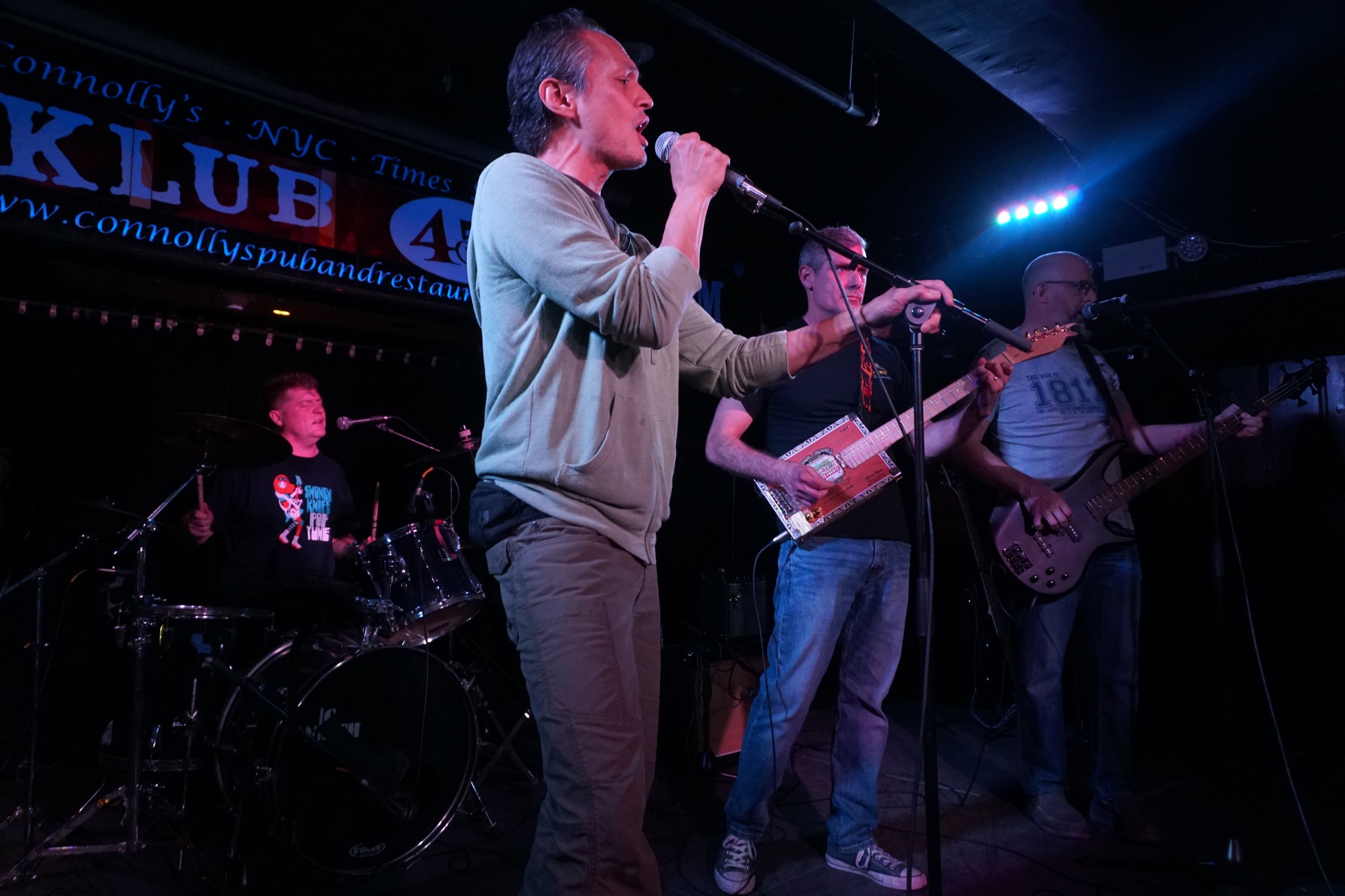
[1088,797,1119,836]
[1027,791,1089,838]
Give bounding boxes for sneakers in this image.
[825,843,927,890]
[714,832,757,895]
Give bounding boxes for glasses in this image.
[1032,280,1098,296]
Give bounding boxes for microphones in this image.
[1082,294,1131,320]
[336,416,396,430]
[655,131,784,211]
[408,477,425,514]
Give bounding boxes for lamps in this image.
[1083,234,1208,281]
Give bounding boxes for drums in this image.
[214,634,480,871]
[117,607,299,842]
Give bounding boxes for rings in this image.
[993,376,997,380]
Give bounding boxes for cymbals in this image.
[154,412,290,474]
[87,499,177,536]
[75,566,142,580]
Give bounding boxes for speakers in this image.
[659,574,771,765]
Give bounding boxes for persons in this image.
[183,372,378,649]
[706,224,1013,893]
[467,6,954,895]
[949,253,1269,838]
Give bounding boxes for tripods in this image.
[670,621,762,808]
[1,453,212,896]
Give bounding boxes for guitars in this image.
[752,324,1078,540]
[995,353,1329,595]
[338,517,486,647]
